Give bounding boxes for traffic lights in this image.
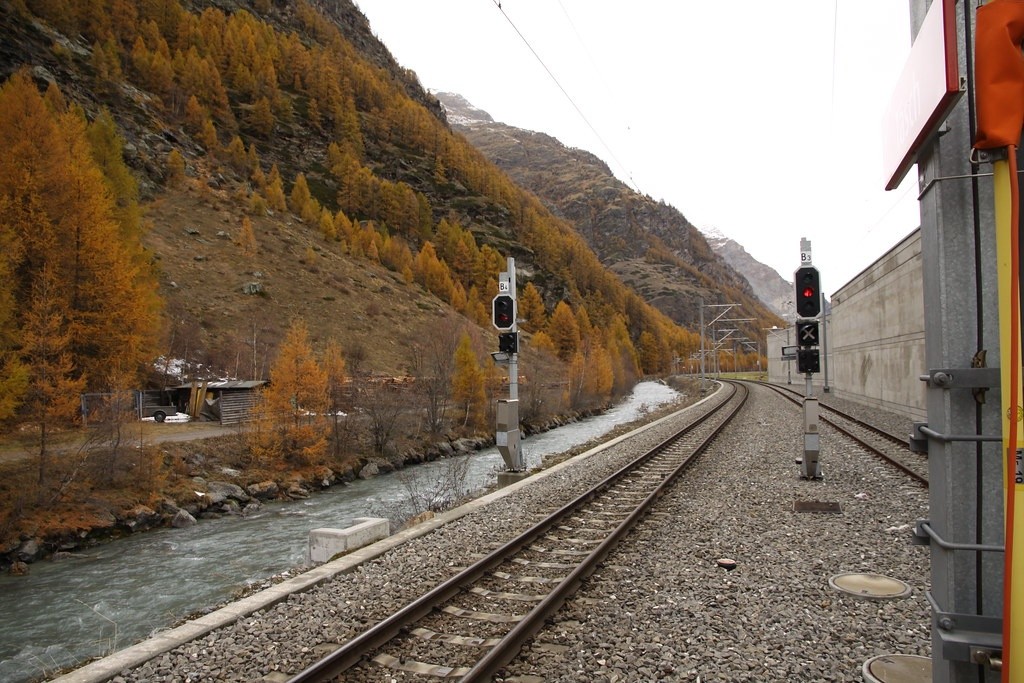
[794,266,822,373]
[492,294,519,354]
